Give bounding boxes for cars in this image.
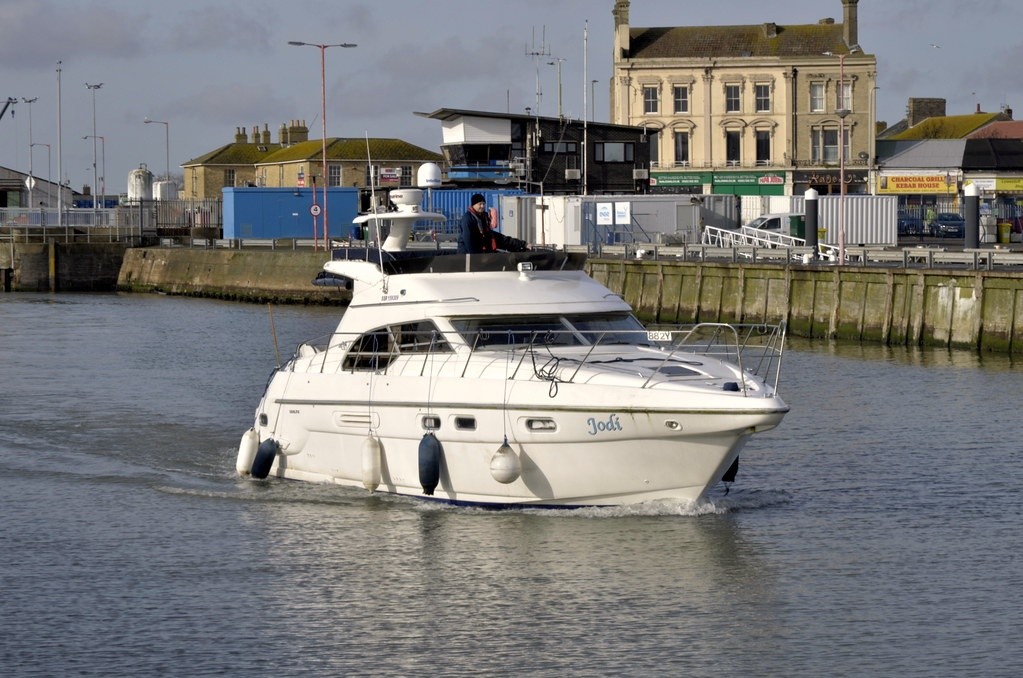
[898,206,923,235]
[930,211,966,238]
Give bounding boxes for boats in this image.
[235,101,789,516]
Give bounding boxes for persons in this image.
[457,194,497,254]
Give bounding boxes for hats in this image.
[471,194,486,206]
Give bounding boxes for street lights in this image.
[822,47,863,264]
[54,58,65,224]
[20,95,42,211]
[29,140,52,211]
[286,40,359,250]
[84,80,98,211]
[144,116,171,202]
[81,135,106,208]
[590,78,601,122]
[545,58,563,118]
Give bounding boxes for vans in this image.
[734,213,804,244]
